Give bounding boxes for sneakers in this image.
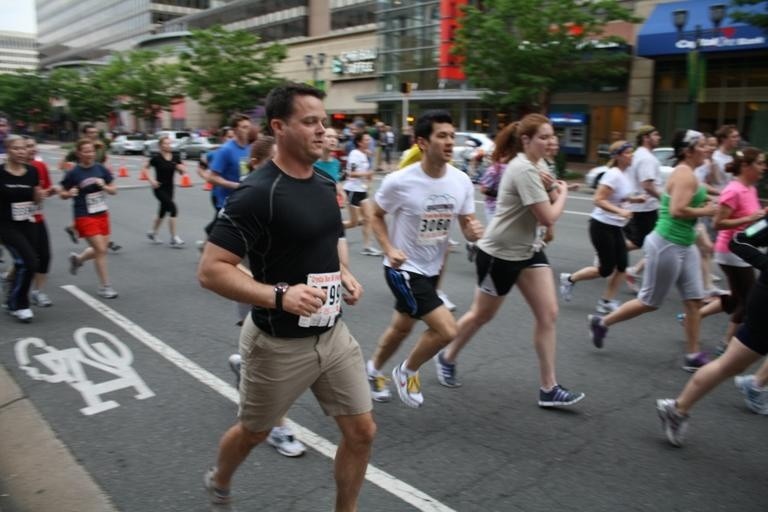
[539,385,585,407]
[229,354,241,388]
[367,360,391,402]
[391,363,422,408]
[265,427,307,457]
[2,227,120,320]
[560,267,729,373]
[362,243,381,255]
[193,241,205,262]
[144,232,162,244]
[204,472,230,504]
[734,375,767,415]
[656,398,688,446]
[467,242,478,263]
[168,238,187,248]
[433,349,462,387]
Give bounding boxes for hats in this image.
[666,129,702,158]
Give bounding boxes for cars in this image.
[177,137,225,162]
[109,132,148,158]
[447,133,496,170]
[585,147,691,192]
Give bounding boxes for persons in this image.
[229,348,306,458]
[195,112,558,251]
[365,113,482,412]
[435,110,586,411]
[562,127,768,439]
[0,83,767,512]
[0,114,56,321]
[55,121,124,298]
[195,84,377,510]
[139,137,185,248]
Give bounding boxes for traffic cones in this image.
[136,167,150,181]
[177,173,193,188]
[117,166,129,177]
[203,180,213,191]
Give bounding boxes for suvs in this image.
[144,129,193,159]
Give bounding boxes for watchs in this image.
[274,281,288,312]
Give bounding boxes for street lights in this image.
[672,4,728,133]
[304,51,326,89]
